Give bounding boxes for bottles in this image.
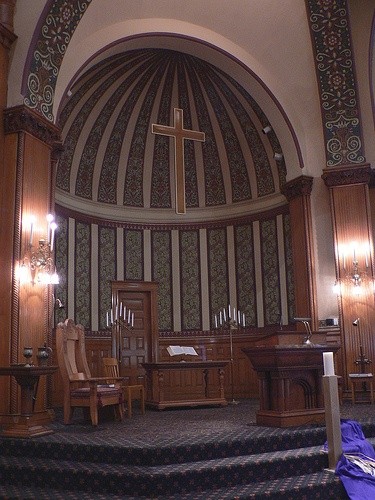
[38,347,53,366]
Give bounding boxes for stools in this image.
[349,373,374,406]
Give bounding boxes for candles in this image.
[116,306,118,318]
[107,312,108,326]
[215,305,246,328]
[124,306,126,320]
[131,313,134,326]
[120,301,122,316]
[111,309,113,323]
[128,309,130,323]
[324,352,334,375]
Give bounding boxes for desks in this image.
[0,366,58,438]
[140,360,230,410]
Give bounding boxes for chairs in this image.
[102,358,144,419]
[56,319,130,426]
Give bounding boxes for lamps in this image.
[334,247,369,295]
[18,214,63,288]
[262,126,272,135]
[273,152,284,160]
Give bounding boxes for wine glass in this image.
[23,347,34,367]
